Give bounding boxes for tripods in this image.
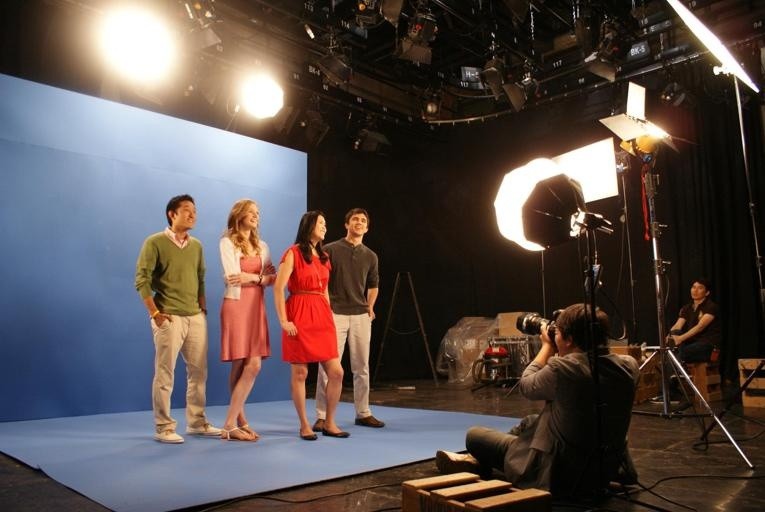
[636,165,758,470]
[370,271,440,386]
[502,255,548,398]
[545,224,673,511]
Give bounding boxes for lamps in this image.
[490,158,615,507]
[599,81,755,470]
[667,0,765,443]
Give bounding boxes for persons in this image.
[272,211,350,441]
[436,303,639,502]
[647,280,721,404]
[218,198,277,442]
[135,194,225,443]
[313,207,384,431]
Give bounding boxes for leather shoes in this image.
[299,429,318,440]
[322,428,351,437]
[313,419,325,432]
[355,416,384,427]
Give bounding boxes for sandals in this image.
[221,428,256,441]
[240,425,260,440]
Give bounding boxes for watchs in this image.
[256,275,263,285]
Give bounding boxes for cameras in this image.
[516,311,556,337]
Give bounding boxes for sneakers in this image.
[186,425,222,436]
[436,450,492,477]
[154,433,184,444]
[650,397,680,405]
[670,368,693,382]
